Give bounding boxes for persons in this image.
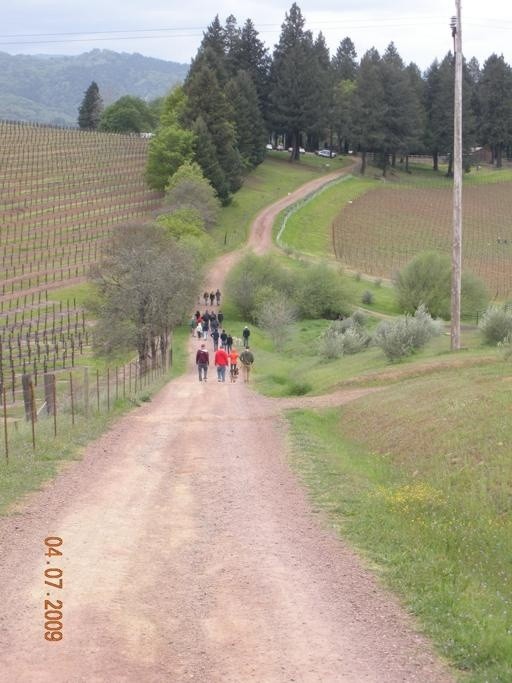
[190,308,234,354]
[242,325,250,349]
[239,346,254,384]
[204,291,209,306]
[215,288,221,306]
[195,344,209,383]
[210,291,215,306]
[214,346,229,383]
[227,349,239,384]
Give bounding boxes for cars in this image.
[288,146,305,153]
[318,150,337,158]
